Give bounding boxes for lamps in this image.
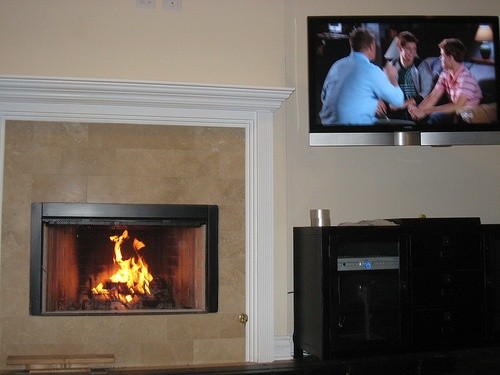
[474,25,494,59]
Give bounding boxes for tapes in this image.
[310,208,330,227]
[393,131,422,147]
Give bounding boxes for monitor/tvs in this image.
[308,15,500,148]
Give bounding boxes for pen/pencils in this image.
[431,145,453,147]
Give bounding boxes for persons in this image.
[375,31,432,116]
[319,28,404,124]
[408,38,483,126]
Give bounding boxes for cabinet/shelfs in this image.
[292,223,500,372]
